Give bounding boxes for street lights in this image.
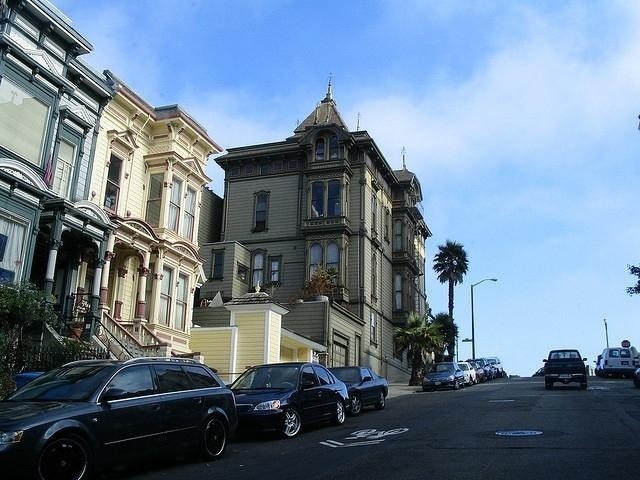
[603,318,609,348]
[471,278,499,358]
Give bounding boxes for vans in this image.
[595,346,638,376]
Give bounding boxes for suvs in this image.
[0,356,238,480]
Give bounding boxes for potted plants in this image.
[70,321,84,338]
[308,265,340,301]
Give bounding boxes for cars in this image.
[226,362,351,438]
[422,357,505,391]
[325,366,389,417]
[633,366,640,389]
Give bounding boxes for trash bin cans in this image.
[15,372,45,390]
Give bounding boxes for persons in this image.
[329,149,338,159]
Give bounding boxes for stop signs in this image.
[621,340,632,349]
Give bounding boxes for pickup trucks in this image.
[542,349,589,389]
